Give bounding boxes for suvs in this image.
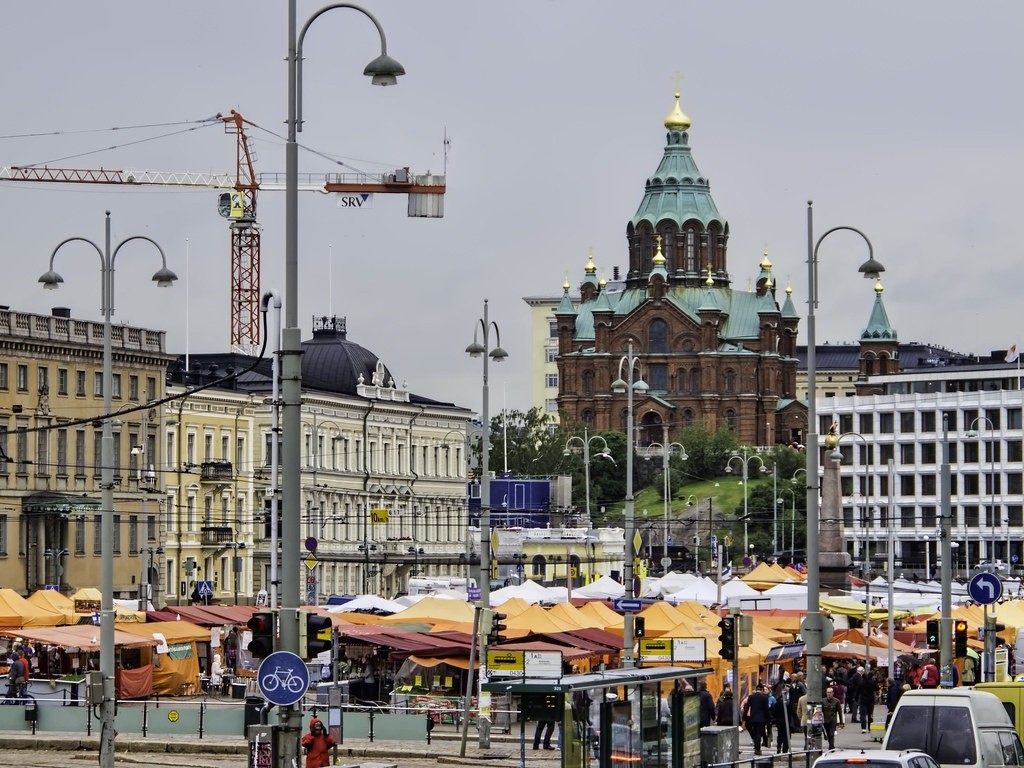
[812,749,941,768]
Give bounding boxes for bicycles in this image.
[0,682,37,707]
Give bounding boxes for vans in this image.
[645,545,700,572]
[881,676,1024,768]
[766,549,806,566]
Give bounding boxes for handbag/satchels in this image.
[920,667,929,682]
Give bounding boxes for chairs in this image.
[201,673,260,696]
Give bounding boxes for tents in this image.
[0,564,1024,702]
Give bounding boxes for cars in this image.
[973,559,1013,573]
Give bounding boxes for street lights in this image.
[686,494,698,571]
[777,488,795,564]
[299,409,345,540]
[965,416,997,683]
[643,442,689,576]
[829,432,871,675]
[610,338,650,669]
[110,399,180,603]
[38,209,178,768]
[43,548,70,593]
[805,199,886,768]
[562,426,612,583]
[465,298,509,749]
[276,0,407,768]
[724,450,767,575]
[439,429,501,593]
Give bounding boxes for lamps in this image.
[93,420,103,428]
[13,405,23,412]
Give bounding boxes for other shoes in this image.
[851,720,860,723]
[848,710,852,713]
[765,742,767,747]
[533,746,538,749]
[754,749,762,755]
[544,745,555,750]
[862,729,866,733]
[762,741,765,746]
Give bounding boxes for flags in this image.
[722,561,731,580]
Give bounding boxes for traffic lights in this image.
[246,611,276,659]
[718,617,734,659]
[955,620,968,658]
[489,612,507,643]
[305,613,334,659]
[636,617,645,637]
[927,620,939,645]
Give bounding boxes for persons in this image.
[533,720,556,750]
[654,690,671,738]
[3,639,71,706]
[715,654,978,755]
[301,718,336,768]
[698,682,716,728]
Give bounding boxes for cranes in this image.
[0,111,447,355]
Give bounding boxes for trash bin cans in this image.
[245,696,264,740]
[231,683,246,698]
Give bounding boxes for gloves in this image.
[321,726,326,735]
[311,728,315,735]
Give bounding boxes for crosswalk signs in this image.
[198,581,212,595]
[45,585,58,592]
